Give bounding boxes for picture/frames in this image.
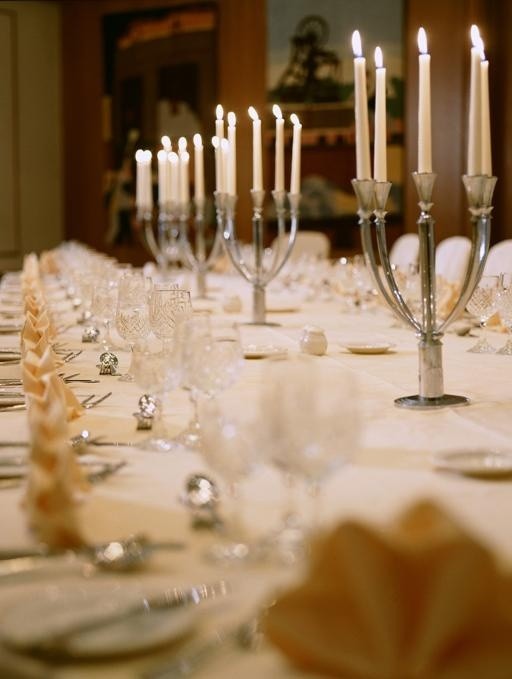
[262,1,408,229]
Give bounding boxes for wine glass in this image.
[55,240,511,555]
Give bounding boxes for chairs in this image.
[388,233,422,271]
[274,231,330,258]
[435,236,473,283]
[483,239,511,292]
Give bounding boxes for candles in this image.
[273,103,285,194]
[289,114,302,195]
[468,25,483,177]
[417,26,433,177]
[192,134,206,217]
[352,28,372,181]
[213,105,237,196]
[250,107,262,191]
[135,132,189,220]
[372,46,388,185]
[478,40,492,174]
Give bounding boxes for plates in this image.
[0,571,196,660]
